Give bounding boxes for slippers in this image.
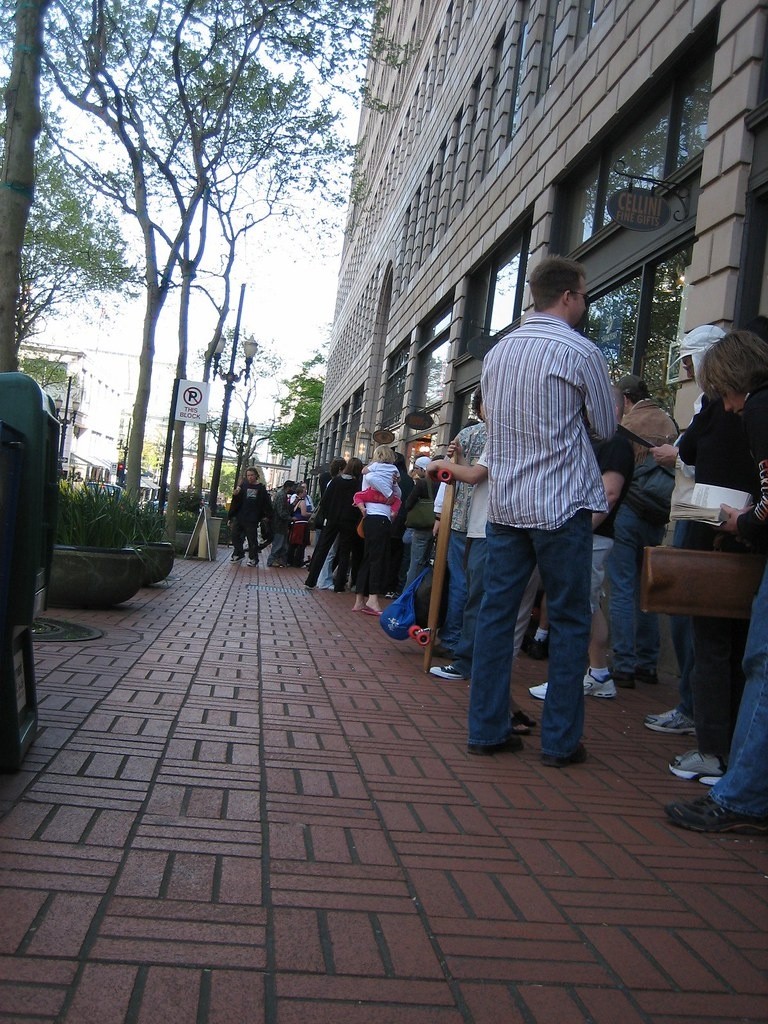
[352,606,382,616]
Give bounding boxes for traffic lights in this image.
[117,464,124,477]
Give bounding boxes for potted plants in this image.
[43,475,165,615]
[128,496,174,586]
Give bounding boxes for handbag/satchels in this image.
[289,523,307,545]
[380,565,433,641]
[308,506,324,531]
[404,478,435,529]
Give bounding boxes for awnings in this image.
[73,453,113,469]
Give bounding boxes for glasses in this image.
[682,362,693,373]
[562,288,590,308]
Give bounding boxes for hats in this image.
[668,325,726,393]
[618,372,643,393]
[416,457,432,471]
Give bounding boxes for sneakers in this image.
[668,751,729,786]
[583,668,617,698]
[528,682,548,700]
[665,794,768,836]
[643,708,697,737]
[429,664,466,680]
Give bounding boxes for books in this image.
[668,484,752,527]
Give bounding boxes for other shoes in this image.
[384,590,402,599]
[611,670,634,688]
[229,554,356,594]
[633,665,658,685]
[432,643,454,658]
[521,630,549,661]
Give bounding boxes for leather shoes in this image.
[543,744,586,768]
[468,735,524,757]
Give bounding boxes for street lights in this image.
[116,433,129,469]
[209,334,258,516]
[231,418,256,490]
[54,394,80,482]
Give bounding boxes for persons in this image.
[227,314,768,835]
[466,255,616,765]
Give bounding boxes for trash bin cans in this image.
[0,370,60,774]
[198,517,223,561]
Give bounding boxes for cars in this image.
[81,482,122,500]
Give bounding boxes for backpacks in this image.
[617,415,682,528]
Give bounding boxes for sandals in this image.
[511,710,537,735]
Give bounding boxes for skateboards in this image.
[408,441,457,673]
